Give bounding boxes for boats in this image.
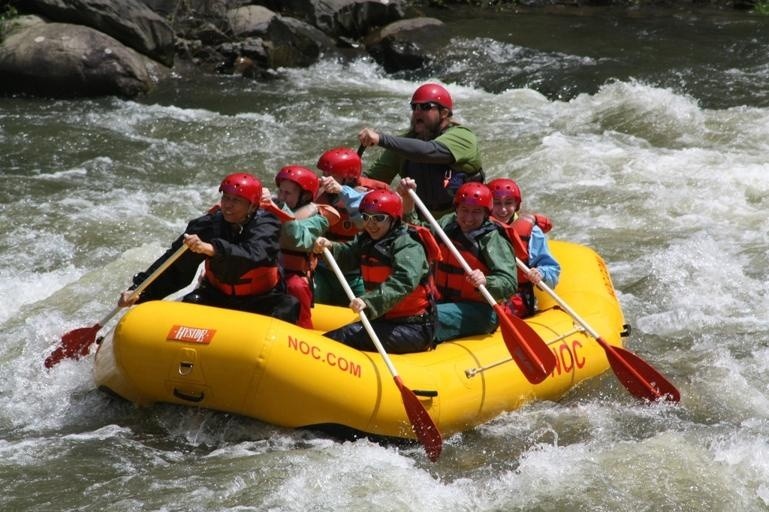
[91,239,632,442]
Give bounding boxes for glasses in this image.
[362,213,389,223]
[411,103,436,110]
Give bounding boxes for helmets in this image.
[411,84,452,110]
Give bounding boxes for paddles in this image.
[42,236,191,369]
[513,254,681,407]
[403,178,558,386]
[320,242,443,463]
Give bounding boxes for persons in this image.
[401,175,520,344]
[312,148,393,308]
[360,82,484,241]
[257,163,338,330]
[314,187,444,355]
[483,179,564,315]
[119,171,299,340]
